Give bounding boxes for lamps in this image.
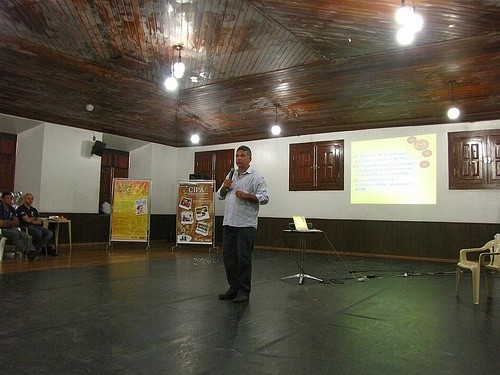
[164,45,185,92]
[271,103,281,136]
[447,79,460,120]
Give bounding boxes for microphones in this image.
[229,168,235,179]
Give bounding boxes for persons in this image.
[216,146,269,302]
[16,192,53,254]
[0,192,42,262]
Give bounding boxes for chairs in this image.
[455,238,500,305]
[0,228,23,262]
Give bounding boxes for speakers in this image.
[91,140,106,156]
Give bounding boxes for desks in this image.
[280,229,324,284]
[42,217,71,255]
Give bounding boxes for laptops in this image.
[293,216,320,231]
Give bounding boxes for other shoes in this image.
[218,289,237,300]
[27,250,40,261]
[232,290,249,302]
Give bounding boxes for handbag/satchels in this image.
[41,240,56,256]
